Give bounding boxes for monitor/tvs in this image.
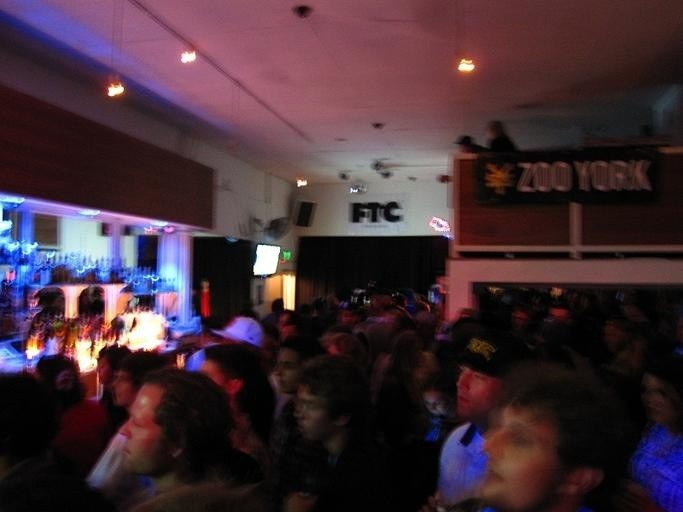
[252,243,282,279]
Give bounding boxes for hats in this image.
[210,316,264,348]
[458,326,508,380]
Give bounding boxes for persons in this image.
[435,134,484,183]
[1,265,683,512]
[485,119,518,150]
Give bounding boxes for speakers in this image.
[295,200,314,227]
